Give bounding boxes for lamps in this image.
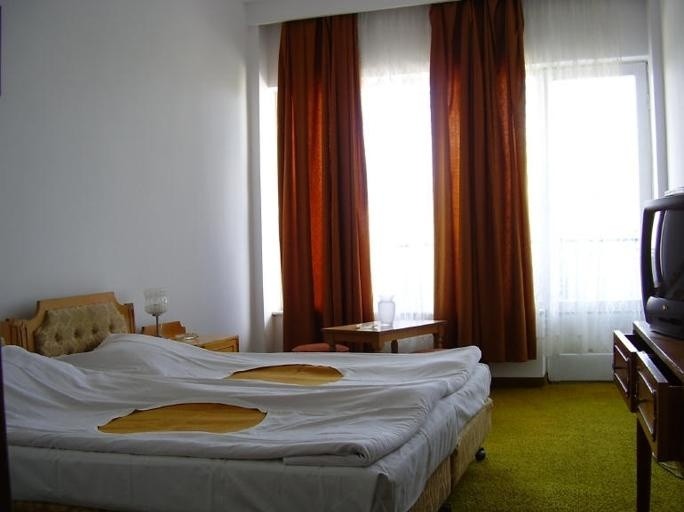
[142,288,167,338]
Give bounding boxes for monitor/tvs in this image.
[640,193,684,340]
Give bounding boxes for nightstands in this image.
[139,322,239,352]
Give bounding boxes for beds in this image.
[0,291,492,511]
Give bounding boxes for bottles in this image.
[379,296,395,324]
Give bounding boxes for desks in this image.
[320,320,448,353]
[612,321,684,512]
